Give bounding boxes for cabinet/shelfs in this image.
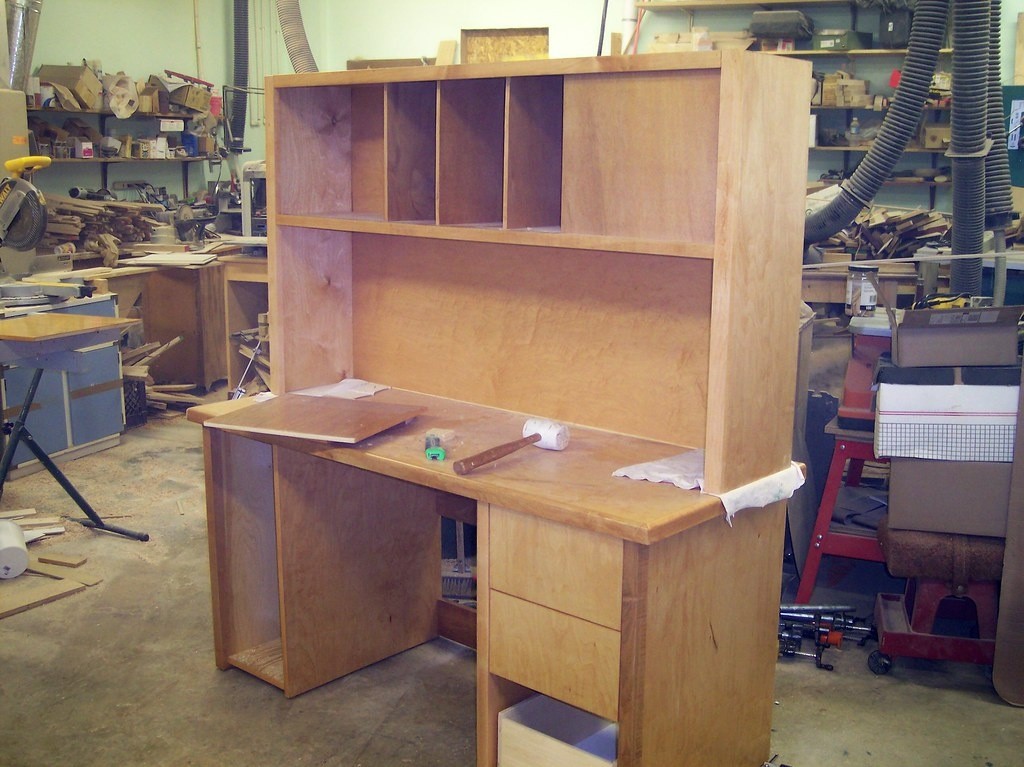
[0,292,126,481]
[25,105,229,198]
[186,48,814,767]
[632,0,954,210]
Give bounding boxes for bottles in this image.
[850,117,859,147]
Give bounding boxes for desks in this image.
[795,415,889,604]
[0,313,149,542]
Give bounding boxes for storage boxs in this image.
[848,306,905,359]
[812,28,873,50]
[498,693,617,767]
[917,122,951,149]
[27,63,215,159]
[888,457,1012,537]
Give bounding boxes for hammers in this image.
[452,417,571,476]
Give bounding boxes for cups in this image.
[53,242,76,254]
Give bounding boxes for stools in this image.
[867,516,1004,674]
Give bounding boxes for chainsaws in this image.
[0,177,47,252]
